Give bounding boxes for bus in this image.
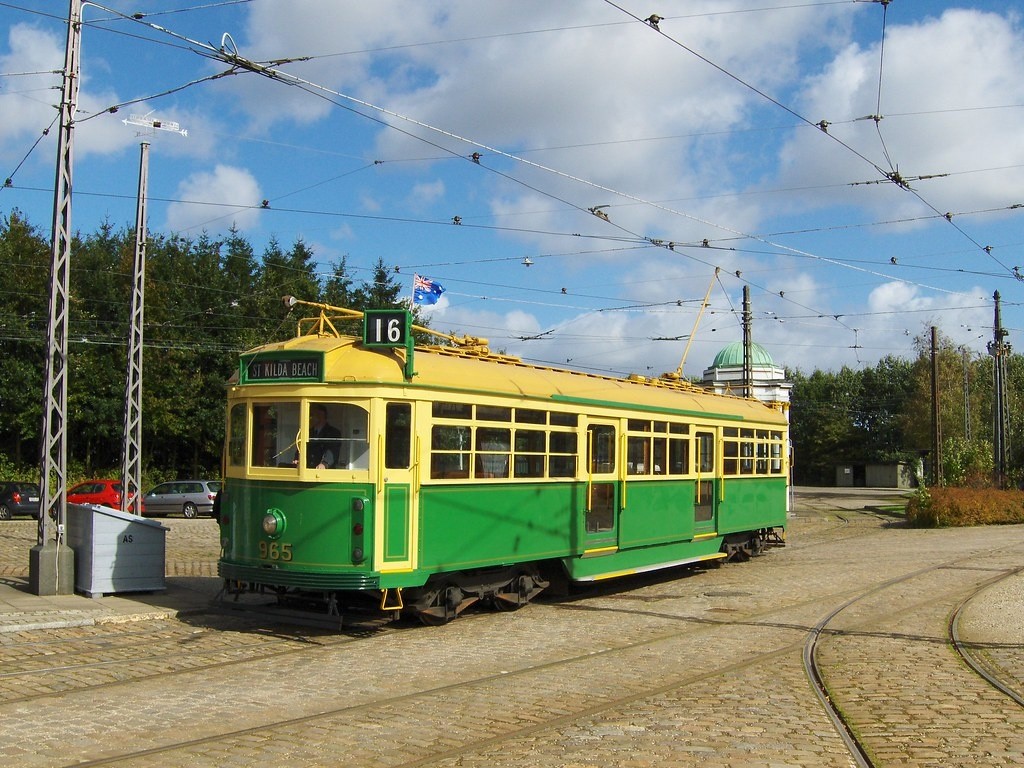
[213,297,790,627]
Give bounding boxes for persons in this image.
[291,403,342,469]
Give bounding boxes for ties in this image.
[314,429,318,438]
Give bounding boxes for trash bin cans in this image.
[69,503,171,599]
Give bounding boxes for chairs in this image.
[186,485,196,493]
[95,486,102,494]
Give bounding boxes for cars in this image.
[0,481,41,521]
[142,481,219,520]
[48,481,143,521]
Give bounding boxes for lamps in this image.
[903,329,911,336]
[230,302,240,309]
[521,258,534,267]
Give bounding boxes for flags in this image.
[414,273,446,305]
